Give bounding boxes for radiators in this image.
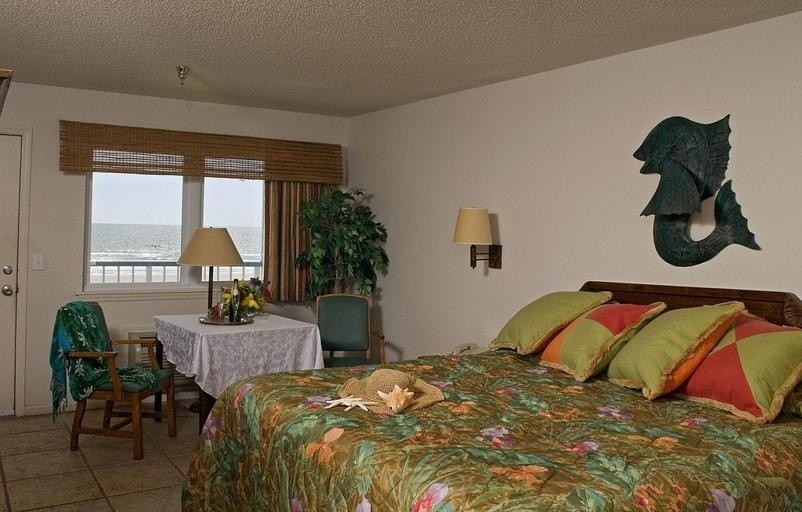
[128,332,194,385]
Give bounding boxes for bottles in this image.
[229,278,241,322]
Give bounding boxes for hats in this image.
[337,368,445,415]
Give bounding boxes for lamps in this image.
[176,228,244,314]
[454,208,502,269]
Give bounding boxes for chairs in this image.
[62,301,176,460]
[316,294,385,368]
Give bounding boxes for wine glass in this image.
[231,293,240,322]
[217,292,226,321]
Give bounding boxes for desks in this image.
[155,313,324,436]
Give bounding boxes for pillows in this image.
[534,302,665,382]
[673,309,802,425]
[488,291,613,355]
[604,301,744,400]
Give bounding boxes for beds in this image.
[182,281,802,512]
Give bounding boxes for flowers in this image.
[207,277,273,321]
